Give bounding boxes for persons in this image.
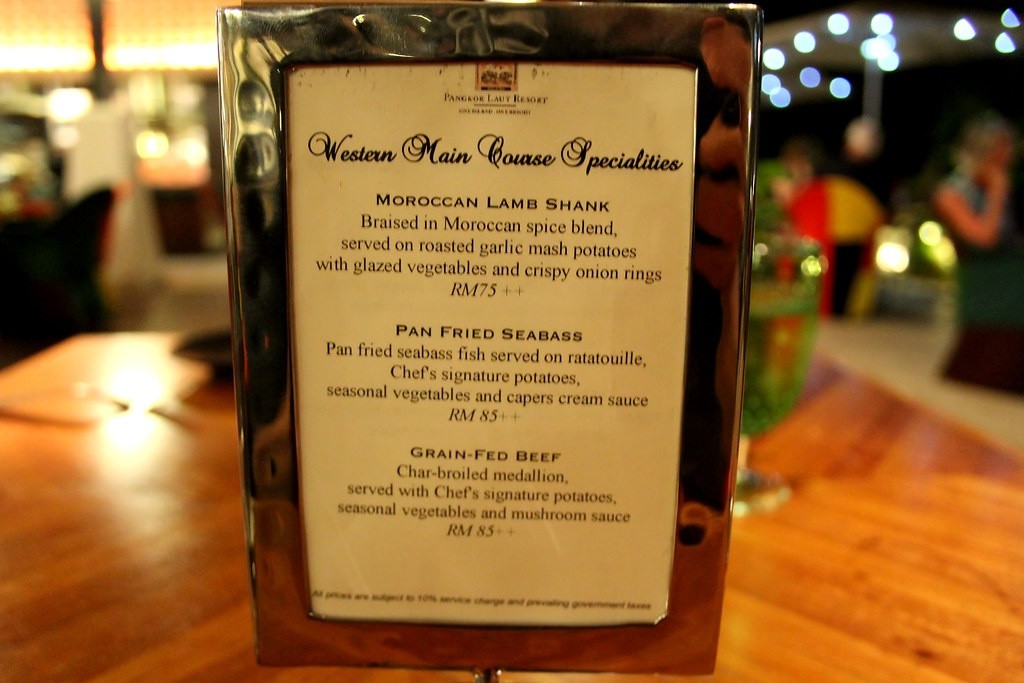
[931,114,1024,397]
[826,112,894,315]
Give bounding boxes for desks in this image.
[0,331,1024,683]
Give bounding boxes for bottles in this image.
[205,0,766,682]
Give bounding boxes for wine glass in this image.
[727,232,822,515]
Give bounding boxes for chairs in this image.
[0,187,115,355]
